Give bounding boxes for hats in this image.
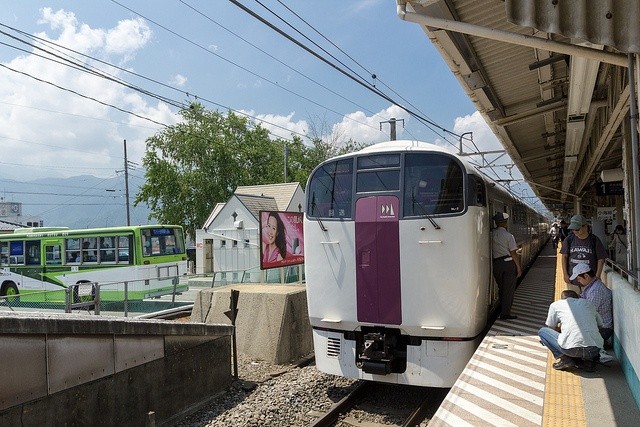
[560,221,566,224]
[567,214,587,229]
[493,213,509,223]
[569,262,591,280]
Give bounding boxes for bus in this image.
[0,224,191,312]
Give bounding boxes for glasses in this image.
[571,229,579,231]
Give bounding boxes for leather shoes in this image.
[552,359,574,369]
[500,315,517,320]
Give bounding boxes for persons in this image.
[549,222,560,250]
[101,237,108,256]
[261,211,287,262]
[559,221,568,247]
[612,233,627,263]
[568,263,612,340]
[94,239,98,256]
[83,241,90,254]
[491,212,522,320]
[560,216,605,296]
[538,289,615,371]
[612,225,626,236]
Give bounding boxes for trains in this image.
[302,139,554,392]
